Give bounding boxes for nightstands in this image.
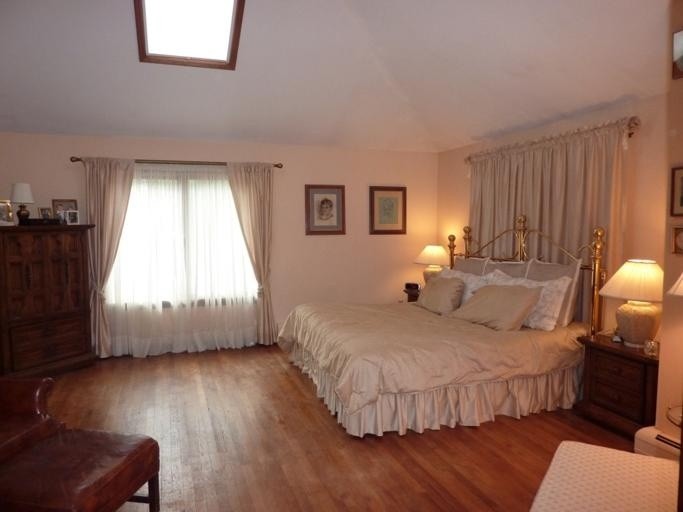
[403,289,421,302]
[573,332,659,441]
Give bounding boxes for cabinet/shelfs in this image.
[0,223,101,378]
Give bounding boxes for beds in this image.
[318,214,606,453]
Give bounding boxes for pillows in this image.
[447,285,545,333]
[525,257,583,328]
[451,258,489,277]
[486,269,572,331]
[415,276,462,319]
[438,268,491,306]
[483,260,528,279]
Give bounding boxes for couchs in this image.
[0,428,160,511]
[0,375,66,465]
[528,440,680,512]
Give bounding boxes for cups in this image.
[645,339,658,356]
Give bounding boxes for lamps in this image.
[9,183,34,223]
[413,244,450,284]
[133,0,245,72]
[599,258,664,348]
[667,270,682,296]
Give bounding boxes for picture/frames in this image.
[368,186,407,236]
[670,166,683,217]
[304,183,347,236]
[37,208,53,222]
[51,199,78,222]
[670,225,683,255]
